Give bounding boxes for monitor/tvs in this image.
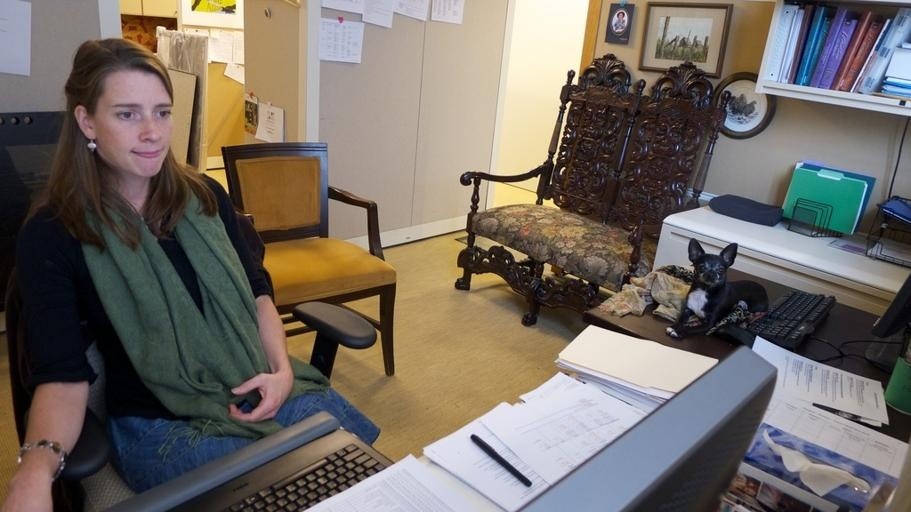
[516,345,777,512]
[864,272,911,374]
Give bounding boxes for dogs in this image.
[664,238,769,340]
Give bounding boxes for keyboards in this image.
[164,429,397,512]
[749,290,836,352]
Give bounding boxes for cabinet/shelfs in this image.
[754,0,911,117]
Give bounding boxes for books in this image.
[782,161,911,268]
[763,0,911,99]
[717,390,911,512]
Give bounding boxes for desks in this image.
[654,204,910,320]
[99,325,910,512]
[579,268,909,420]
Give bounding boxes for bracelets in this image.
[13,438,70,484]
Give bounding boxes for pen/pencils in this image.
[471,434,532,488]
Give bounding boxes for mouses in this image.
[716,325,756,347]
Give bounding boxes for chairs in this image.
[6,276,376,510]
[221,142,397,376]
[454,52,731,326]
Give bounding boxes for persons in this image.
[613,12,625,31]
[0,37,381,512]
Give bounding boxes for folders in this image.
[763,4,800,82]
[880,198,911,226]
[782,160,876,240]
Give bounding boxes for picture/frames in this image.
[638,2,733,79]
[714,73,775,140]
